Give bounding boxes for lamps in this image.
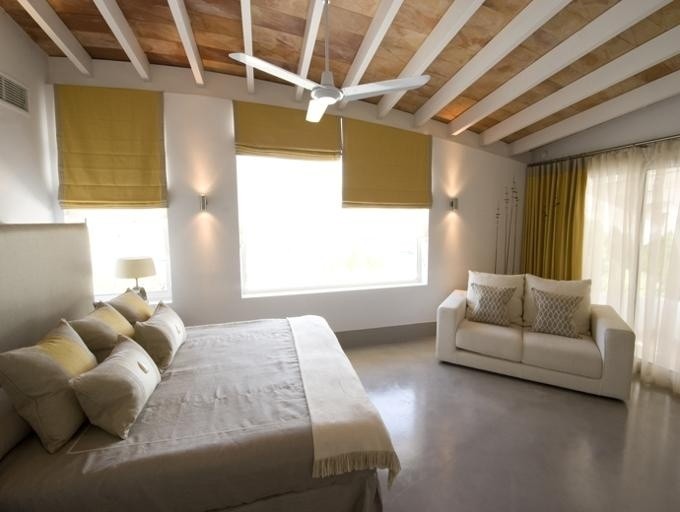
[113,257,156,290]
[199,191,208,213]
[450,195,460,210]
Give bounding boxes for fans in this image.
[229,0,433,123]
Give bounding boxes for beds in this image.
[0,222,383,512]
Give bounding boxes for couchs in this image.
[436,288,636,402]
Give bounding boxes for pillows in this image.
[0,288,188,455]
[464,270,592,339]
